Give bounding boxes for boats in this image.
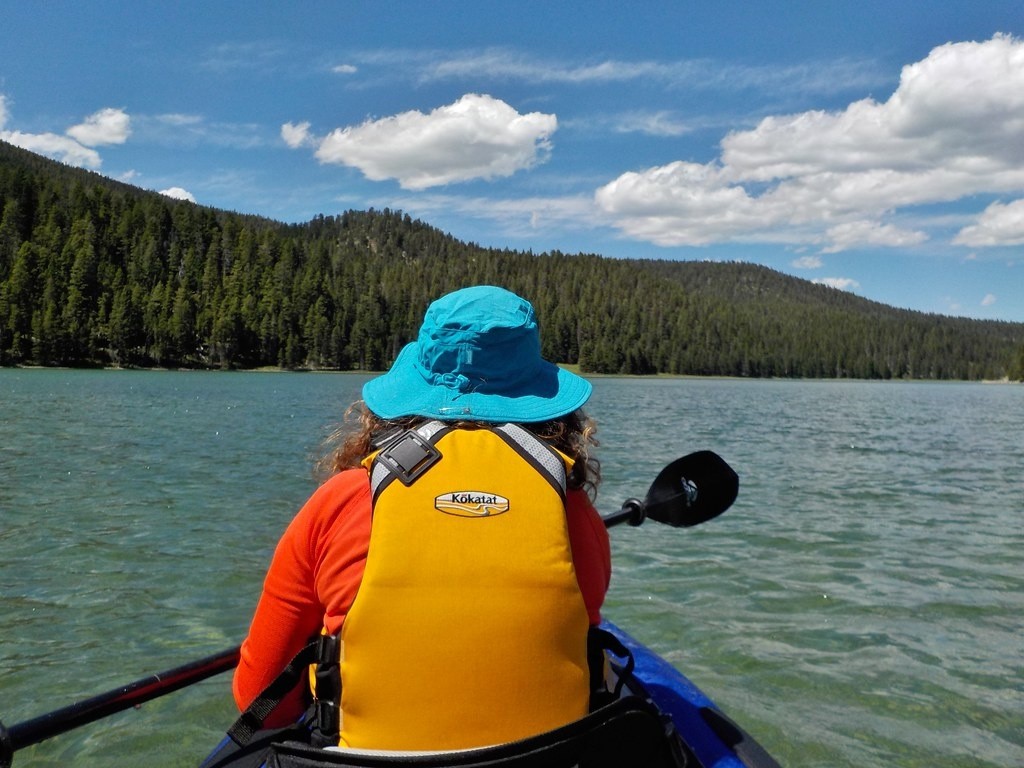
[197,611,782,768]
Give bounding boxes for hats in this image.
[361,285,593,424]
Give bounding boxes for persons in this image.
[203,284,616,766]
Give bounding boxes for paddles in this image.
[1,447,741,768]
